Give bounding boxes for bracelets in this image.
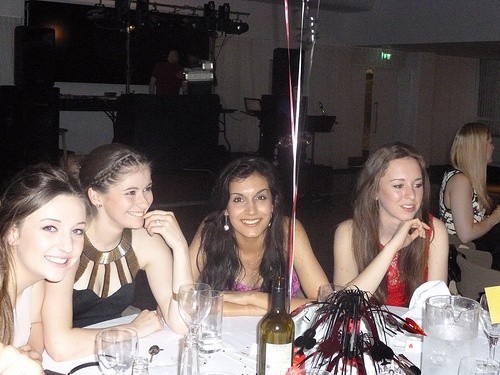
[171,288,194,303]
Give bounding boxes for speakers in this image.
[13,26,56,86]
[272,48,305,97]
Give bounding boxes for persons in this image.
[333,142,449,305]
[148,49,188,96]
[189,155,335,316]
[0,142,195,375]
[439,121,500,301]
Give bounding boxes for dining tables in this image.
[31,296,500,375]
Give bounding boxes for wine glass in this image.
[317,285,348,339]
[177,283,211,333]
[479,294,500,371]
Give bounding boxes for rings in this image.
[156,219,160,224]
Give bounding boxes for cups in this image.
[458,355,500,375]
[95,326,140,375]
[420,295,482,375]
[196,290,224,355]
[280,369,331,375]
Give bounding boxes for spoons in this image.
[148,345,159,362]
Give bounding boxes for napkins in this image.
[398,280,454,325]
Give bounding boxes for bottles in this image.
[256,276,295,375]
[132,357,149,375]
[177,335,199,375]
